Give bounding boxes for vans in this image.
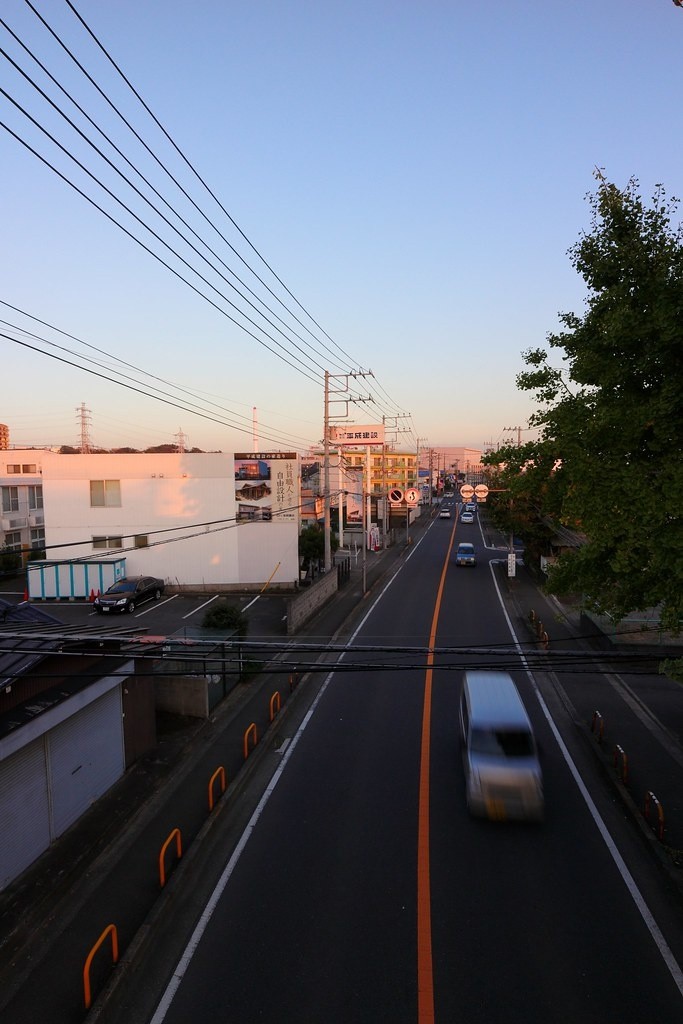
[456,543,476,567]
[458,670,545,820]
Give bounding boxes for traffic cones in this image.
[90,589,94,603]
[24,587,29,602]
[97,589,100,598]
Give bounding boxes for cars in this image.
[462,496,477,511]
[94,575,165,614]
[460,512,474,524]
[440,508,451,518]
[443,492,454,497]
